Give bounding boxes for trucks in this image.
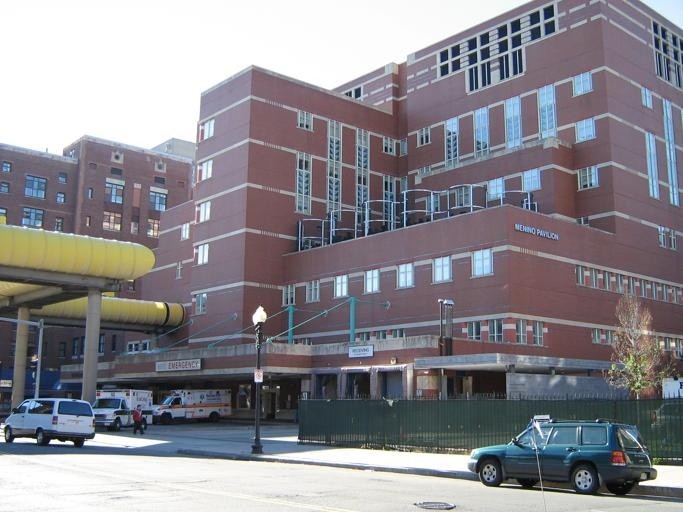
[91,388,153,431]
[152,388,232,424]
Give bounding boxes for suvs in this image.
[466,415,656,495]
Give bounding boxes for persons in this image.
[133,405,145,434]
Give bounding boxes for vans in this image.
[3,397,95,447]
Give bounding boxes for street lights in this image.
[251,304,267,454]
